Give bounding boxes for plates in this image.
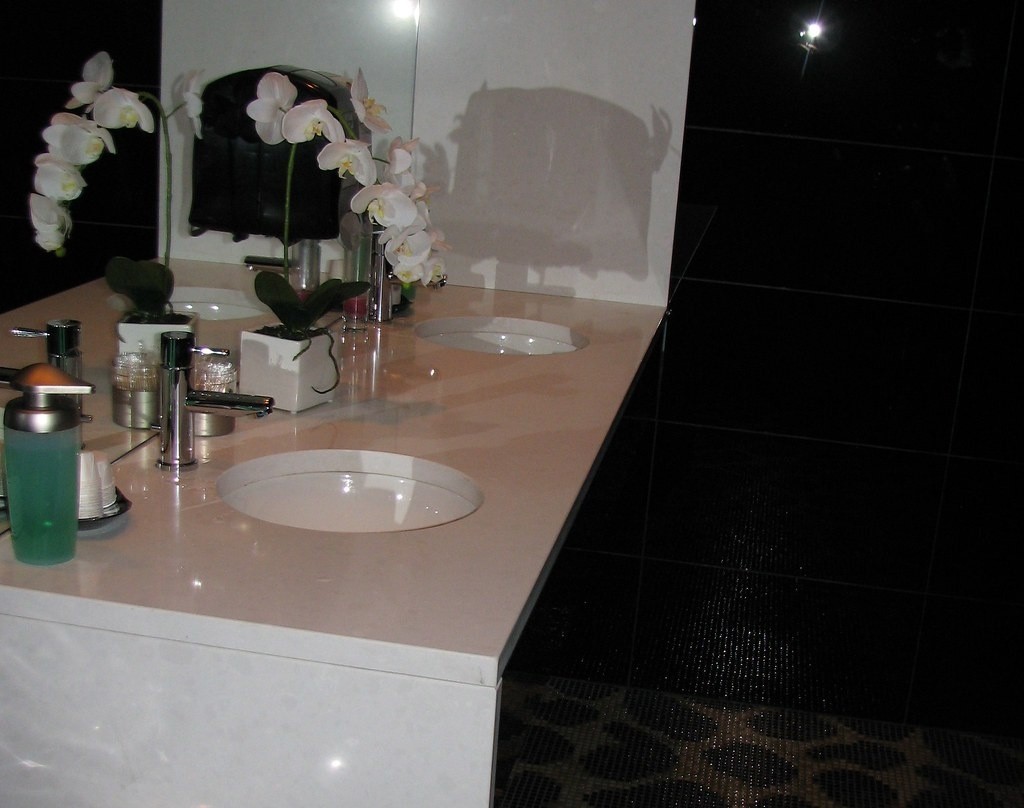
[78,486,132,523]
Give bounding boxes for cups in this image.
[112,388,159,428]
[192,411,240,436]
[94,450,117,508]
[79,452,103,519]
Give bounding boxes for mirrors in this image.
[0,0,419,537]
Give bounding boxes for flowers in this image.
[29,48,209,342]
[247,58,451,390]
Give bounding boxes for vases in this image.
[238,323,341,416]
[113,308,199,368]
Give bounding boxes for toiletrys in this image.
[1,361,97,567]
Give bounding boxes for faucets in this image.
[0,320,92,451]
[148,331,276,473]
[243,239,321,331]
[368,219,448,323]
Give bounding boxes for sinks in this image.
[214,449,485,534]
[104,286,267,322]
[413,316,591,356]
